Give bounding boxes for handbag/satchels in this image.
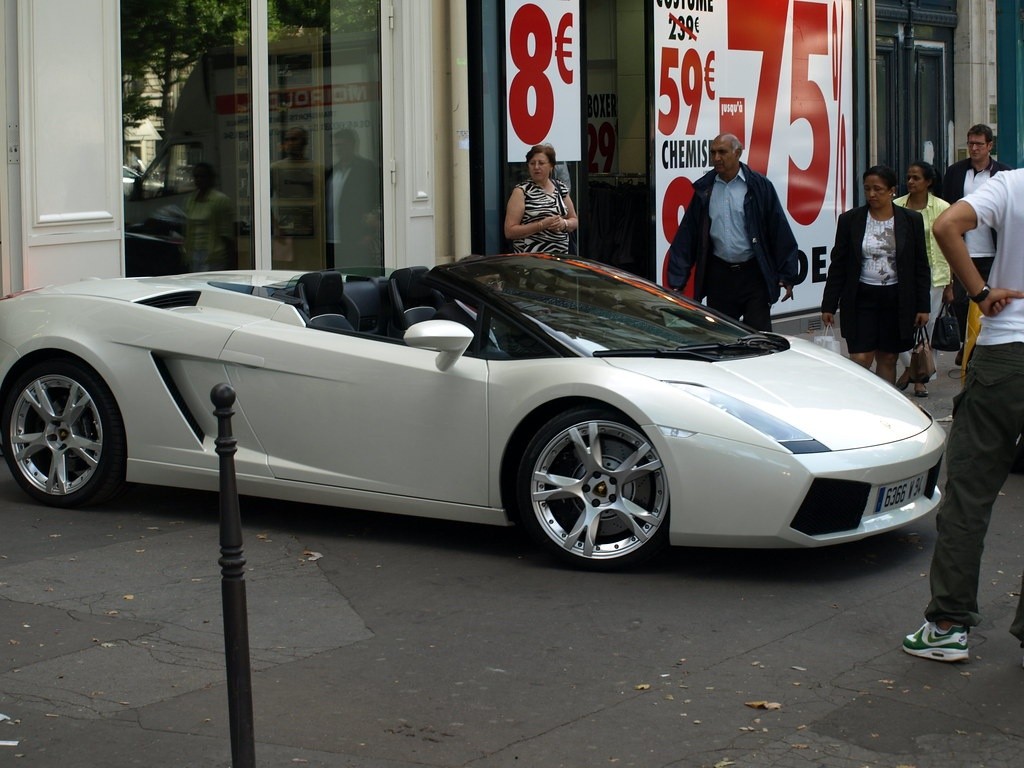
[908,320,937,384]
[567,238,577,257]
[814,322,840,355]
[930,301,961,352]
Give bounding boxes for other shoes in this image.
[896,367,912,391]
[954,342,976,366]
[913,382,928,397]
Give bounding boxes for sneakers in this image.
[901,617,968,662]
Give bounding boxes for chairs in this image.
[294,273,362,331]
[386,266,481,347]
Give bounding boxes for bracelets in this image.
[562,219,569,233]
[966,284,990,302]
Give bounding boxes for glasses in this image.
[967,141,988,148]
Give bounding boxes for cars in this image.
[124,168,164,202]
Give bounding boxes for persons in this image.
[554,161,571,193]
[270,126,324,269]
[323,129,383,269]
[903,167,1024,662]
[181,163,236,272]
[942,124,1010,366]
[821,166,930,383]
[892,161,952,396]
[431,253,523,357]
[667,133,797,332]
[505,145,577,253]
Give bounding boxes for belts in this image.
[720,261,749,274]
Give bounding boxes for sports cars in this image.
[0,250,953,576]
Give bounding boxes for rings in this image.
[557,228,559,231]
